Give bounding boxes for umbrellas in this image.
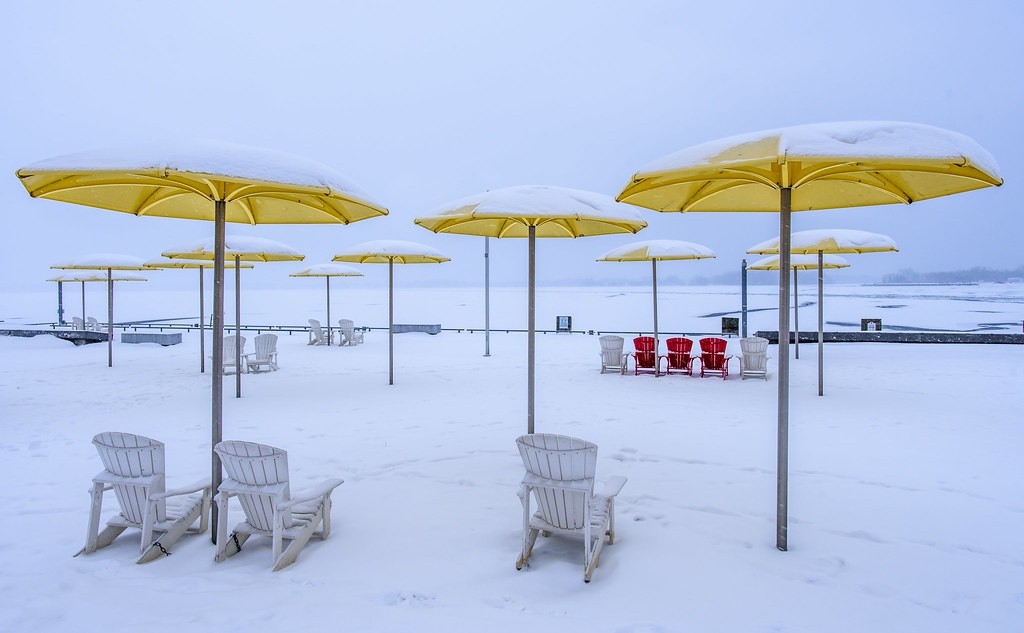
[745,254,851,359]
[50,254,164,367]
[414,185,648,434]
[746,228,900,394]
[331,240,451,383]
[161,235,305,398]
[615,120,1005,548]
[45,270,148,341]
[595,239,717,339]
[143,255,254,373]
[289,264,365,346]
[15,138,389,545]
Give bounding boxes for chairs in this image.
[71,316,84,330]
[86,316,108,332]
[632,336,667,377]
[208,335,247,375]
[665,337,697,376]
[599,335,632,376]
[214,441,344,572]
[736,336,772,381]
[73,432,227,564]
[516,432,627,583]
[338,319,365,346]
[240,333,281,372]
[306,318,336,345]
[697,337,733,380]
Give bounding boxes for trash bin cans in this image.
[721,317,740,337]
[556,316,572,334]
[861,318,882,331]
